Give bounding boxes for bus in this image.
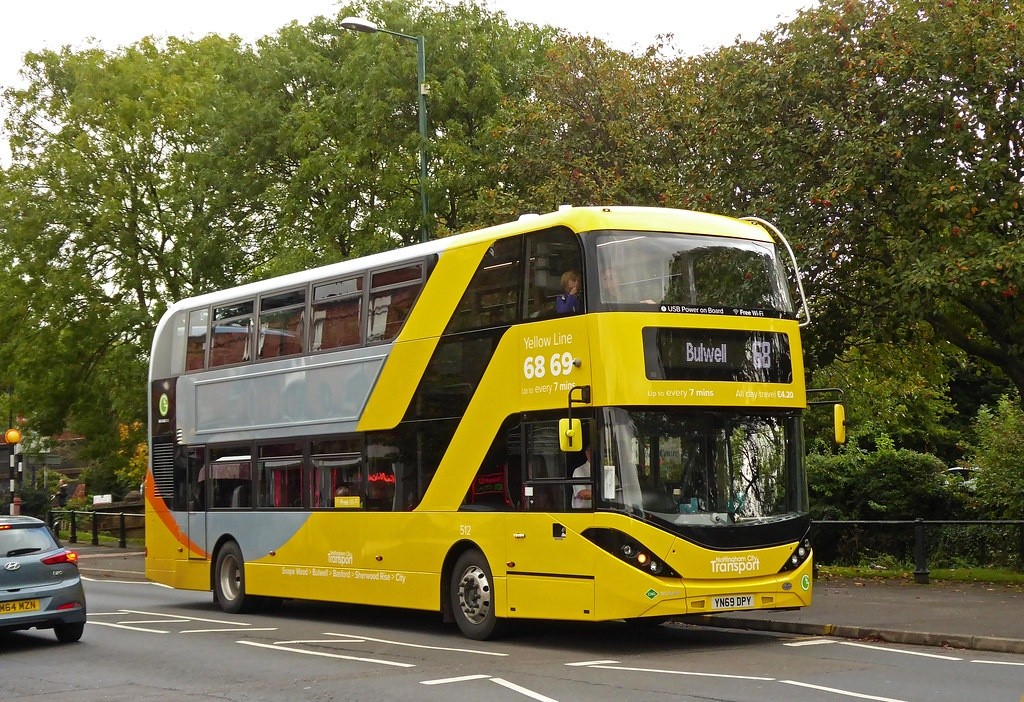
[144,203,852,642]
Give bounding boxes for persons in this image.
[55,478,69,507]
[571,444,622,507]
[555,270,582,315]
[600,266,625,303]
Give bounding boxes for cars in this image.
[939,466,983,492]
[0,514,88,644]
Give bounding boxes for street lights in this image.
[338,16,431,243]
[4,428,22,517]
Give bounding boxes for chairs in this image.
[232,486,246,507]
